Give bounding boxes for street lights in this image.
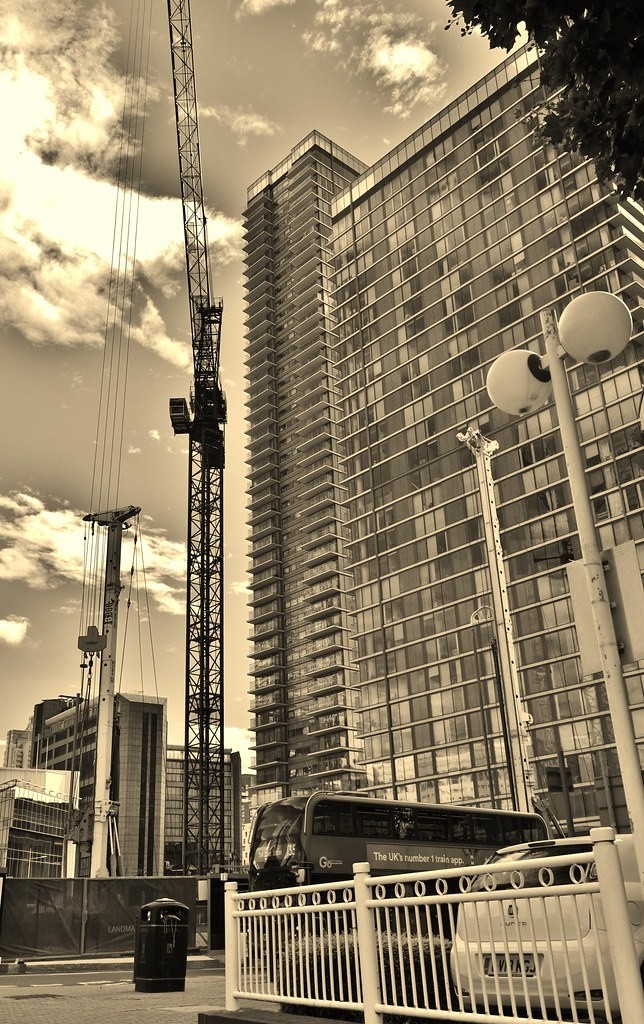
[487,291,644,883]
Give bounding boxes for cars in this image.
[450,833,644,1019]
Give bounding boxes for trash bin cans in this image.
[132,898,190,992]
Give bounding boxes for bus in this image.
[248,790,550,941]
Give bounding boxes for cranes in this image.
[167,0,262,876]
